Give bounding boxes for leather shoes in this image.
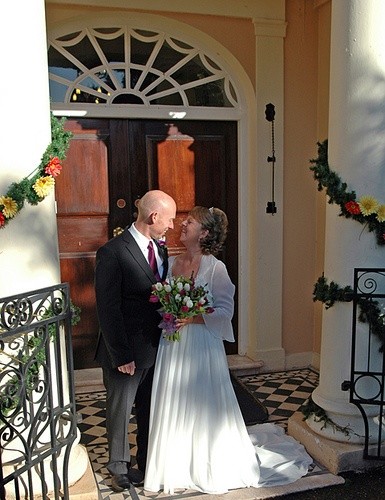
[129,467,144,485]
[111,473,131,492]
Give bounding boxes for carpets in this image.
[229,368,269,426]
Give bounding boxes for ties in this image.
[147,241,161,284]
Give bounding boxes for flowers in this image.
[148,276,214,333]
[0,212,4,227]
[376,203,385,222]
[345,201,359,215]
[0,195,18,218]
[357,194,379,217]
[45,156,62,177]
[31,175,56,199]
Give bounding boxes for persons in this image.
[144,206,260,494]
[93,190,176,492]
